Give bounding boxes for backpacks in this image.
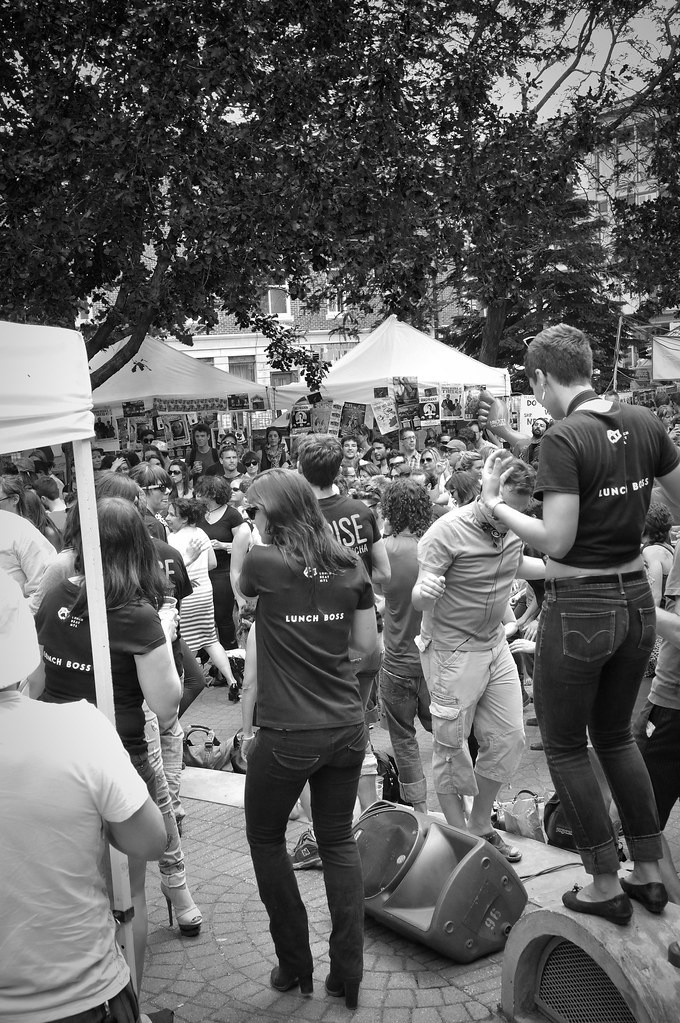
[543,791,627,863]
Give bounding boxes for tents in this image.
[274,315,511,409]
[90,334,267,406]
[0,319,138,1023]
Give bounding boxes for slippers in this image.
[476,830,522,863]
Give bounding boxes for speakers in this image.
[352,800,529,965]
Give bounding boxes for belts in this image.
[544,570,645,592]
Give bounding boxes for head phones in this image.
[474,495,507,539]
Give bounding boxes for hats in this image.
[0,569,41,692]
[15,457,36,473]
[149,440,170,455]
[91,445,104,456]
[440,426,476,452]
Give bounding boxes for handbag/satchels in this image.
[182,724,234,771]
[504,788,545,845]
[372,746,402,804]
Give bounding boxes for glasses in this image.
[141,482,173,493]
[246,505,260,520]
[450,488,457,496]
[116,466,129,473]
[399,436,418,441]
[222,441,235,446]
[167,470,182,476]
[142,438,155,444]
[419,458,434,464]
[245,460,258,467]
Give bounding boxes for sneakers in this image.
[286,831,324,870]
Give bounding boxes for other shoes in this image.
[562,883,633,926]
[145,1007,174,1023]
[619,877,669,911]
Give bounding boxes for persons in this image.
[170,421,184,437]
[297,412,304,426]
[0,569,167,1023]
[424,389,431,397]
[667,943,680,968]
[0,391,680,935]
[94,418,115,439]
[442,394,459,416]
[481,324,680,928]
[230,468,378,1010]
[412,456,536,864]
[425,404,434,417]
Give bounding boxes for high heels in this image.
[227,683,241,704]
[161,881,203,937]
[270,950,313,995]
[324,972,360,1009]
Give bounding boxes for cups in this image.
[155,596,177,612]
[194,461,202,473]
